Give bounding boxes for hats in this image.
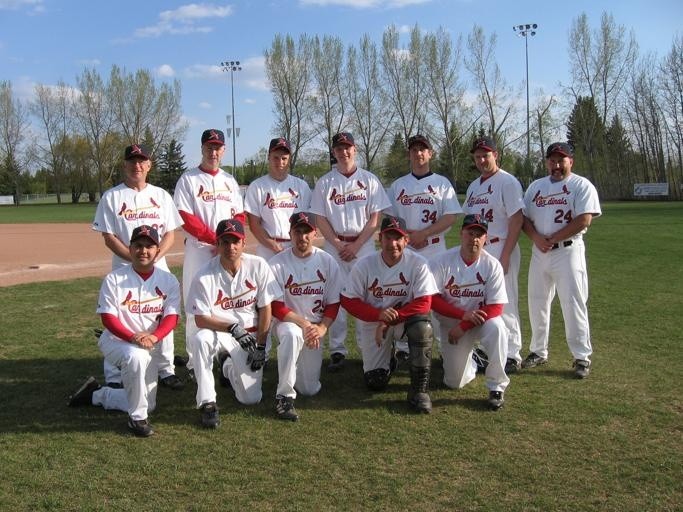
[268,137,291,153]
[470,136,497,153]
[331,133,355,147]
[290,212,316,232]
[130,224,159,246]
[215,219,246,240]
[124,144,150,161]
[380,217,408,236]
[461,213,490,233]
[201,129,225,146]
[545,142,573,158]
[408,135,432,150]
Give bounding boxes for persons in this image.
[267,212,340,420]
[382,136,464,369]
[460,137,524,375]
[244,138,317,370]
[174,129,246,386]
[308,133,392,372]
[340,216,440,414]
[522,142,602,379]
[65,226,181,437]
[185,220,283,429]
[91,145,182,390]
[428,215,511,409]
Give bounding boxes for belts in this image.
[483,237,500,245]
[270,237,291,243]
[337,234,358,242]
[550,240,574,249]
[424,238,439,244]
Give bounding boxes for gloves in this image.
[229,323,266,370]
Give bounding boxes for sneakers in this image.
[390,341,398,372]
[198,401,221,428]
[406,386,432,414]
[470,347,550,373]
[67,375,101,405]
[488,389,505,408]
[273,393,299,421]
[573,359,592,378]
[127,414,155,438]
[102,350,350,389]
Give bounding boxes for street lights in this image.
[221,61,242,179]
[512,23,539,160]
